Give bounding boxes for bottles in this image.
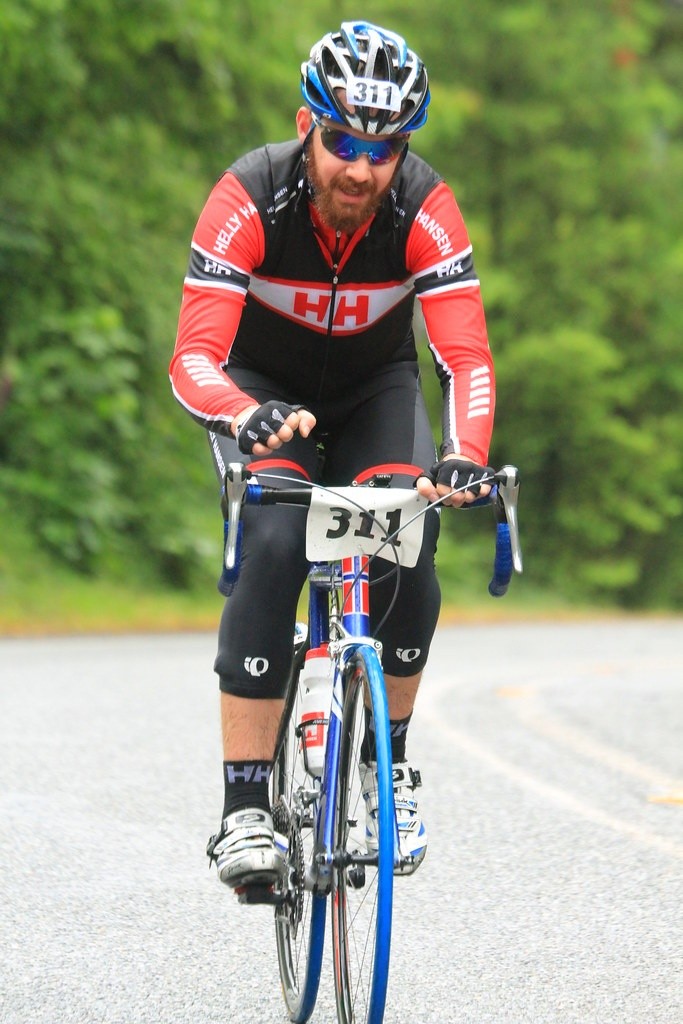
[303,643,336,775]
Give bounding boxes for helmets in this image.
[299,21,430,136]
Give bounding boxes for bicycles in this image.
[220,463,524,1024]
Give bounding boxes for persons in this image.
[170,20,496,904]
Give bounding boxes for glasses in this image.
[321,125,411,166]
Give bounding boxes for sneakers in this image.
[206,806,283,888]
[358,759,427,876]
